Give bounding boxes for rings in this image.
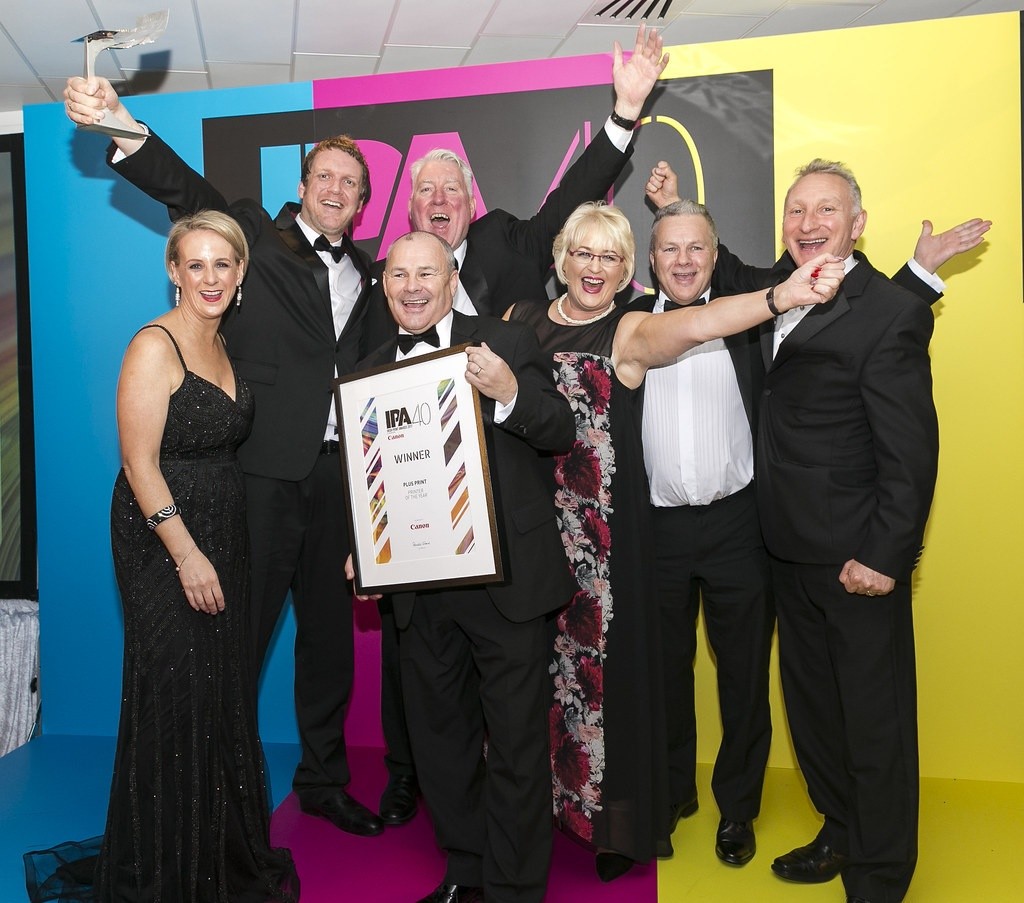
[475,368,482,375]
[69,100,74,111]
[866,590,874,596]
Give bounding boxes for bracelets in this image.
[766,286,789,316]
[146,504,181,529]
[611,109,636,131]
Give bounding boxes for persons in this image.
[99,210,256,903]
[372,26,671,828]
[501,202,845,883]
[608,198,995,867]
[644,160,939,903]
[63,75,383,837]
[331,232,576,903]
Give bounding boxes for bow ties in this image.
[314,234,345,263]
[397,324,440,355]
[664,297,706,312]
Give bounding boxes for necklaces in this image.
[557,292,614,325]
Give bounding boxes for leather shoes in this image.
[416,881,485,903]
[379,772,420,824]
[716,815,756,867]
[299,786,383,835]
[771,829,850,883]
[669,793,699,834]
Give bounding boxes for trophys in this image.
[71,10,170,140]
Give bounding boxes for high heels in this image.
[595,853,635,882]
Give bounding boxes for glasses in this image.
[568,249,625,267]
